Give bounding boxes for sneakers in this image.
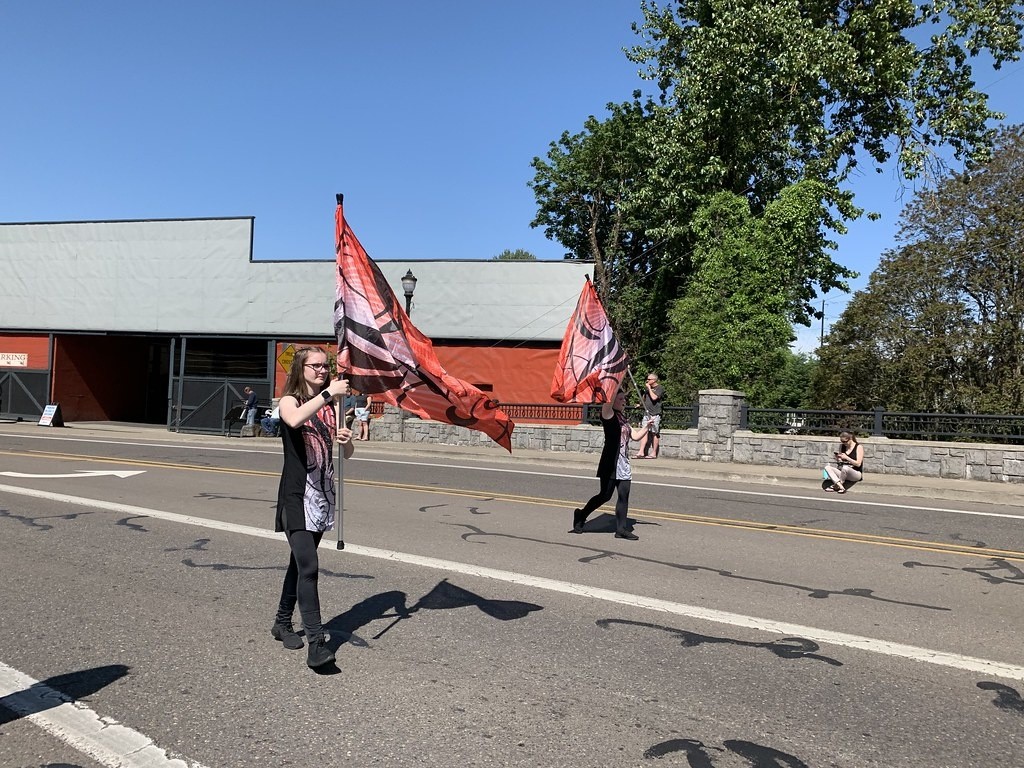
[271,623,304,650]
[306,637,336,667]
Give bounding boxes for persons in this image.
[261,404,279,434]
[822,432,864,494]
[631,372,663,459]
[354,390,372,441]
[270,345,355,667]
[243,386,258,424]
[573,345,654,540]
[344,387,356,424]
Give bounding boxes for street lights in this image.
[401,268,418,319]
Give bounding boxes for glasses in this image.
[842,439,849,443]
[303,363,332,372]
[647,379,655,380]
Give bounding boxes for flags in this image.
[333,204,515,456]
[551,280,630,404]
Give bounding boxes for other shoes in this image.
[354,437,361,440]
[838,489,846,493]
[615,530,639,539]
[361,438,368,441]
[574,508,586,534]
[825,486,836,491]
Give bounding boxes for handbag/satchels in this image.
[240,409,247,419]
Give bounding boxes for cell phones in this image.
[834,452,838,454]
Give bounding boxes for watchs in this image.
[321,391,332,402]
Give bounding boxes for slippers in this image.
[645,455,656,459]
[631,455,644,459]
